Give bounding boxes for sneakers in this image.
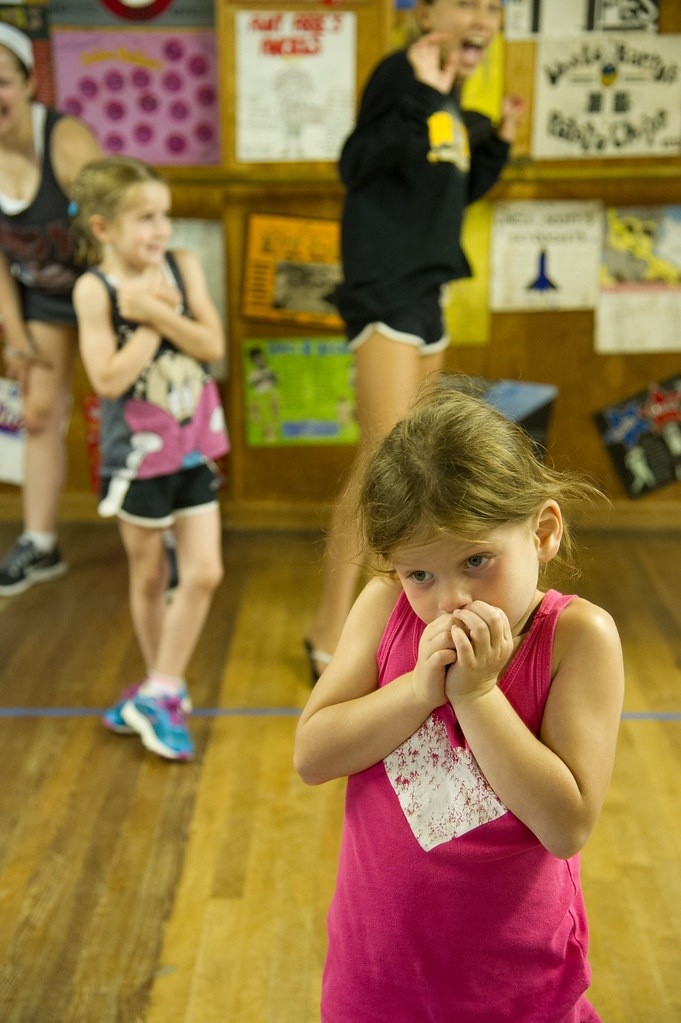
[99,685,140,735]
[120,687,194,761]
[0,537,65,596]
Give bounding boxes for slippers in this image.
[303,637,333,682]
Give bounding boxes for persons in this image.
[291,371,627,1022]
[1,22,184,601]
[303,1,529,681]
[69,158,230,763]
